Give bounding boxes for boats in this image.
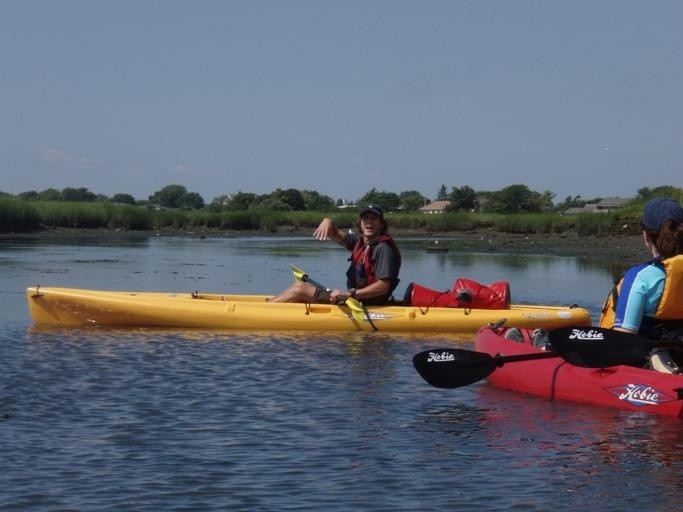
[476,324,683,417]
[27,286,591,333]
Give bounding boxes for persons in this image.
[269,202,403,304]
[596,196,683,336]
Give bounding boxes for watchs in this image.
[347,286,356,298]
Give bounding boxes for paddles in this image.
[548,326,682,368]
[413,347,560,388]
[287,264,364,326]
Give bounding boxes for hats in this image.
[642,197,682,233]
[358,203,382,218]
